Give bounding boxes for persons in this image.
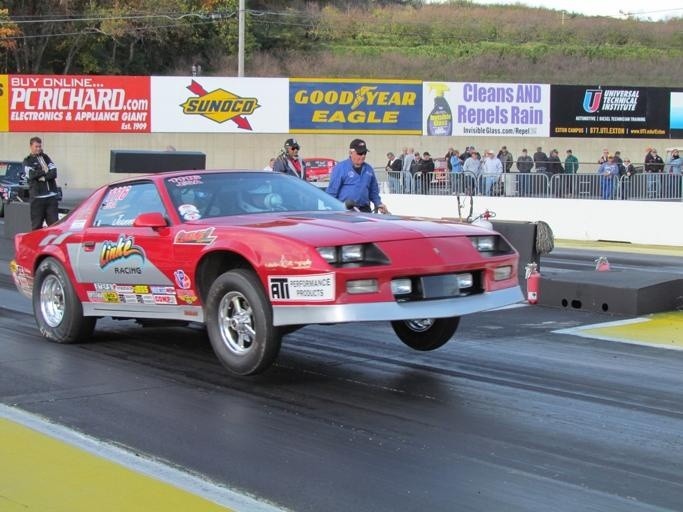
[227,179,287,217]
[272,138,306,182]
[324,138,388,215]
[20,137,59,233]
[383,144,683,202]
[264,158,274,171]
[4,166,19,179]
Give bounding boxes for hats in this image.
[284,138,300,148]
[423,152,431,156]
[608,152,615,158]
[470,149,494,154]
[350,139,369,154]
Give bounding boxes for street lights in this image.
[191,62,202,76]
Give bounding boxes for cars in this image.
[624,163,681,191]
[0,196,4,216]
[429,157,448,185]
[298,156,341,187]
[7,151,530,378]
[0,160,61,204]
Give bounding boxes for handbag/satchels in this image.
[55,186,63,201]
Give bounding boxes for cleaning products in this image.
[425,82,453,136]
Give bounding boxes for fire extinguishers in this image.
[524,261,540,304]
[595,255,610,271]
